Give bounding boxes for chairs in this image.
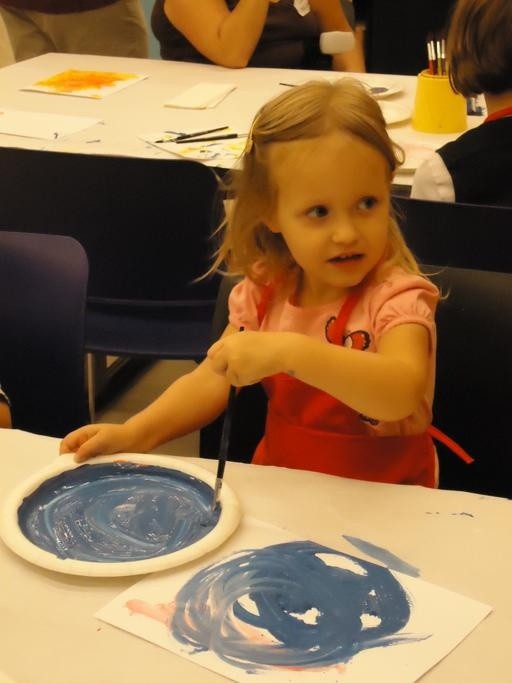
[0,231,90,435]
[395,195,510,271]
[1,147,224,360]
[381,263,510,493]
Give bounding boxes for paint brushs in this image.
[175,132,249,144]
[427,37,435,75]
[212,326,245,513]
[441,32,447,75]
[436,32,442,75]
[154,126,229,144]
[429,32,438,74]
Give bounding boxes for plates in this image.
[0,450,243,577]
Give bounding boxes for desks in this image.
[1,53,486,202]
[0,429,510,683]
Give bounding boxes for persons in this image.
[58,76,442,488]
[0,0,149,62]
[149,0,366,74]
[408,1,511,208]
